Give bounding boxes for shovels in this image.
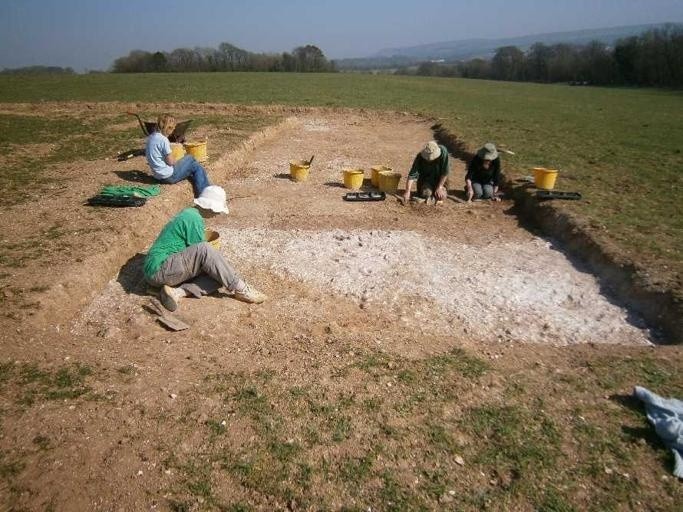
[150,299,189,331]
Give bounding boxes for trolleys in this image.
[127,111,194,142]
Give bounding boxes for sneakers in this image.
[235,284,267,303]
[160,285,180,311]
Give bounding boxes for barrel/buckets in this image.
[289,159,311,181]
[531,165,558,190]
[379,172,401,192]
[170,143,184,161]
[204,227,220,251]
[343,170,364,189]
[372,166,392,187]
[184,142,207,163]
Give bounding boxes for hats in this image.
[420,142,441,161]
[193,184,228,214]
[478,143,498,161]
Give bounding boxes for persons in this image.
[142,185,268,310]
[402,141,449,203]
[465,143,502,203]
[146,113,210,198]
